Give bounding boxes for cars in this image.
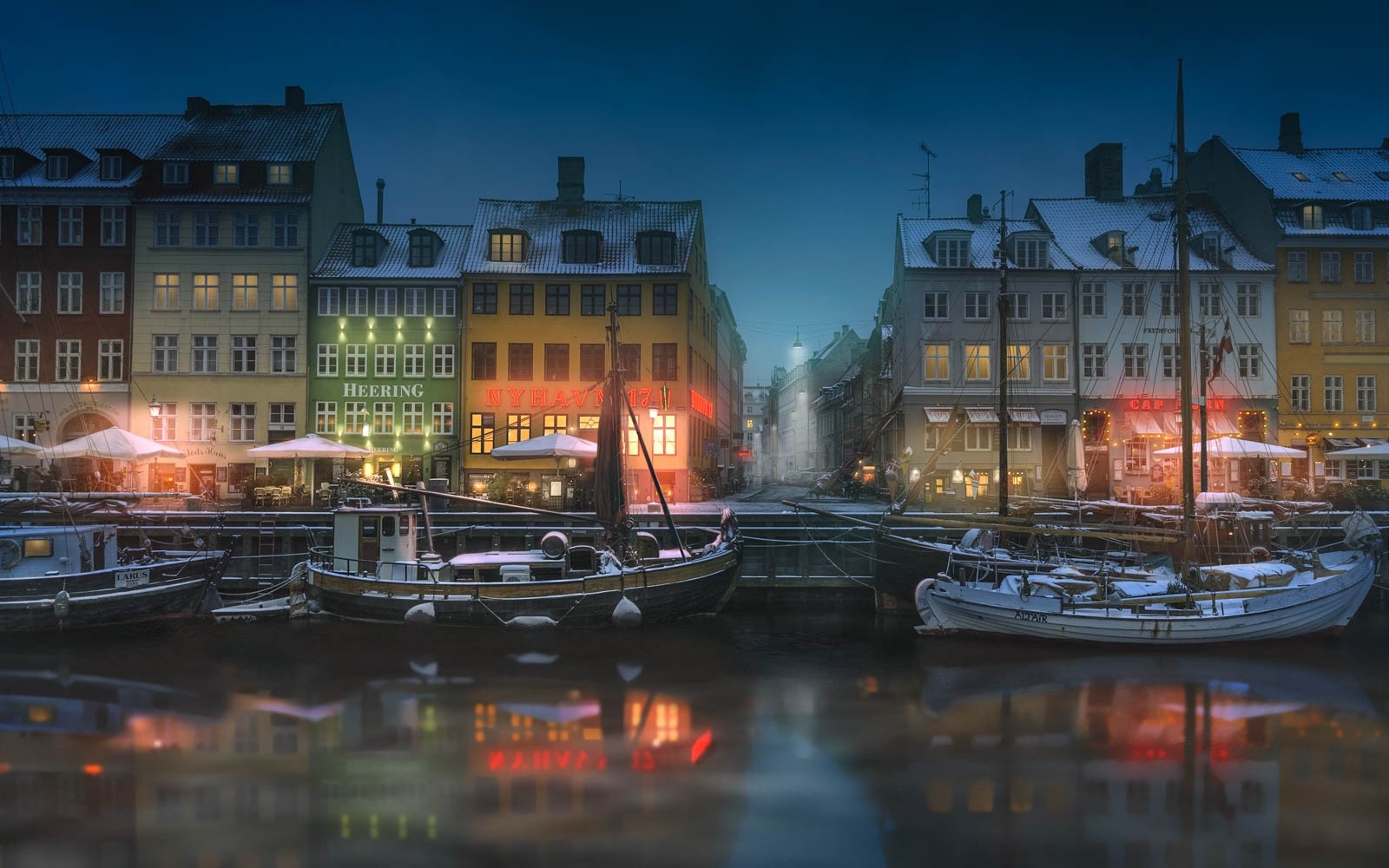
[745,468,832,487]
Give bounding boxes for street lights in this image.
[148,391,162,501]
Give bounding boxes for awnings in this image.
[1007,406,1041,423]
[1326,438,1361,450]
[1357,438,1388,447]
[963,406,1000,423]
[923,407,959,423]
[1124,411,1243,439]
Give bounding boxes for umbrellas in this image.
[0,434,48,456]
[1152,435,1308,494]
[1324,441,1389,477]
[245,433,373,507]
[35,425,185,493]
[1066,418,1088,502]
[490,430,598,477]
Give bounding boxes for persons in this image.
[297,484,306,506]
[882,452,902,505]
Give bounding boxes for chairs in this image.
[1112,486,1153,505]
[505,490,542,507]
[565,544,596,578]
[253,482,333,509]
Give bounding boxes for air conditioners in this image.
[502,573,530,583]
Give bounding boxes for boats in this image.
[297,300,742,629]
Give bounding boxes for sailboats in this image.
[774,51,1388,648]
[0,228,239,634]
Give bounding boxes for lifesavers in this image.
[1246,546,1271,563]
[0,538,21,570]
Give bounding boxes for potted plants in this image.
[242,475,255,508]
